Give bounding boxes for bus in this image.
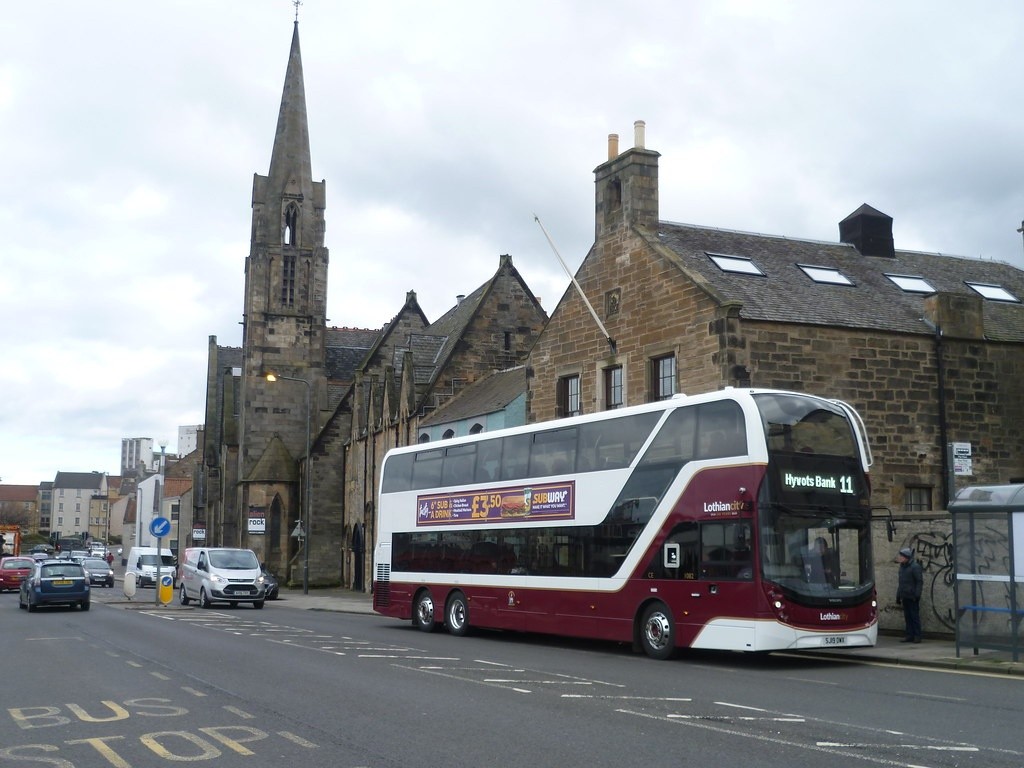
[369,387,894,660]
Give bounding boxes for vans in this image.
[176,547,264,610]
[126,546,176,588]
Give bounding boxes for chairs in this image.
[420,471,440,487]
[497,542,516,571]
[550,458,569,474]
[706,432,727,457]
[512,464,528,479]
[603,457,625,467]
[519,544,537,565]
[531,460,550,477]
[63,567,72,577]
[472,543,495,574]
[575,456,590,472]
[449,468,471,485]
[477,467,490,481]
[493,466,510,480]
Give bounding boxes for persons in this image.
[895,548,923,644]
[810,538,840,581]
[107,553,114,568]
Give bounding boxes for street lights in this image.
[154,437,169,607]
[93,470,110,549]
[263,367,311,596]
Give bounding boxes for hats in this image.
[899,548,913,559]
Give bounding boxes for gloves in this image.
[913,597,919,602]
[896,598,901,605]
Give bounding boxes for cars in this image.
[19,560,91,613]
[261,569,279,601]
[0,541,115,588]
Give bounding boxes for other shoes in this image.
[913,637,921,643]
[900,637,913,642]
